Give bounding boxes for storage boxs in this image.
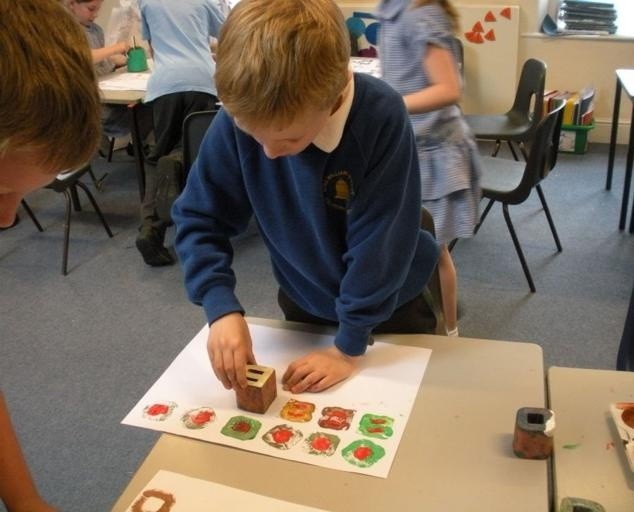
[529,114,595,154]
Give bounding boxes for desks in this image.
[606,69,634,232]
[547,365,634,512]
[95,56,381,202]
[109,314,552,512]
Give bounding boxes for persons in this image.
[132,0,229,267]
[374,0,484,335]
[65,1,154,158]
[171,0,443,392]
[1,0,105,512]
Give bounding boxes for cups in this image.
[125,46,148,72]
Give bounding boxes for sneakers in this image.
[136,225,173,266]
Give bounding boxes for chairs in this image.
[20,161,114,276]
[181,110,221,195]
[447,97,566,294]
[419,205,448,334]
[462,58,547,161]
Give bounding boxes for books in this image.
[542,88,595,125]
